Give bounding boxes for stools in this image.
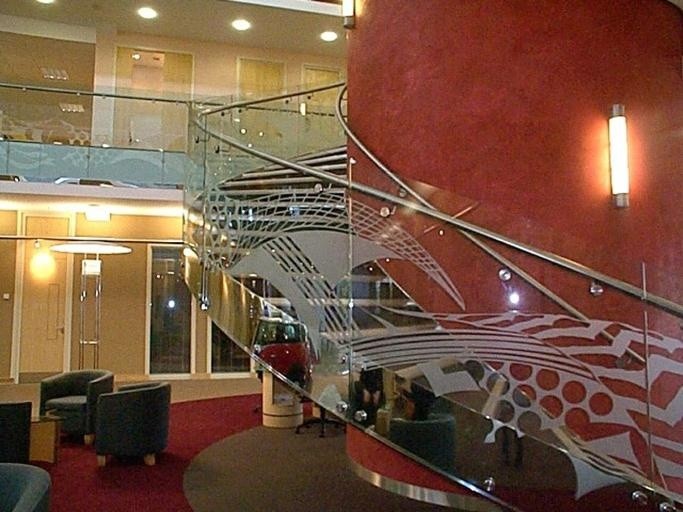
[294,396,347,439]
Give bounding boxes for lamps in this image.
[48,239,134,370]
[606,103,632,211]
[341,0,355,29]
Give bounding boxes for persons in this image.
[392,378,432,422]
[351,361,384,429]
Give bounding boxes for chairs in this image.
[39,369,172,470]
[346,380,386,426]
[388,411,456,474]
